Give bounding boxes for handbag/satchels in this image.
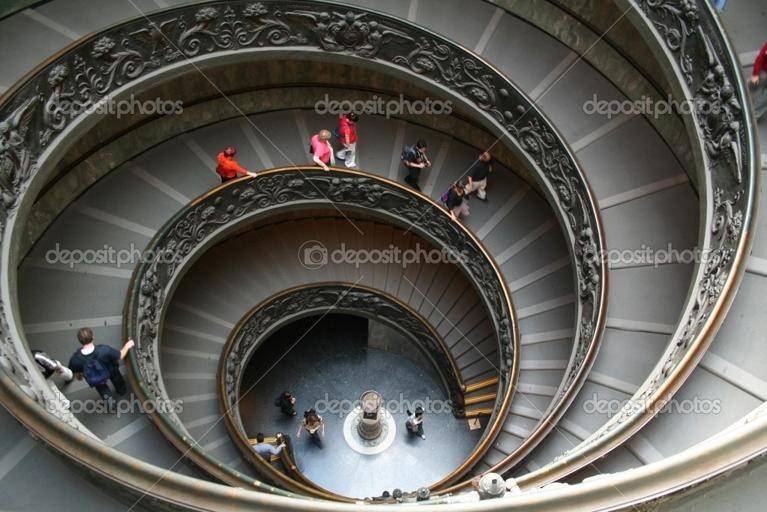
[333,126,340,139]
[441,191,450,202]
[73,346,111,387]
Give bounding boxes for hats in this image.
[347,112,359,123]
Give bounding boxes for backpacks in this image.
[401,144,417,161]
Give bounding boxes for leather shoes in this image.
[477,197,488,202]
[463,191,469,199]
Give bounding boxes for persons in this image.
[68,327,135,409]
[444,181,470,220]
[281,391,297,417]
[336,112,360,168]
[464,151,493,202]
[311,129,335,171]
[216,146,259,183]
[713,0,728,13]
[748,42,767,121]
[31,350,74,412]
[407,407,426,440]
[405,139,431,192]
[296,408,325,450]
[252,433,287,465]
